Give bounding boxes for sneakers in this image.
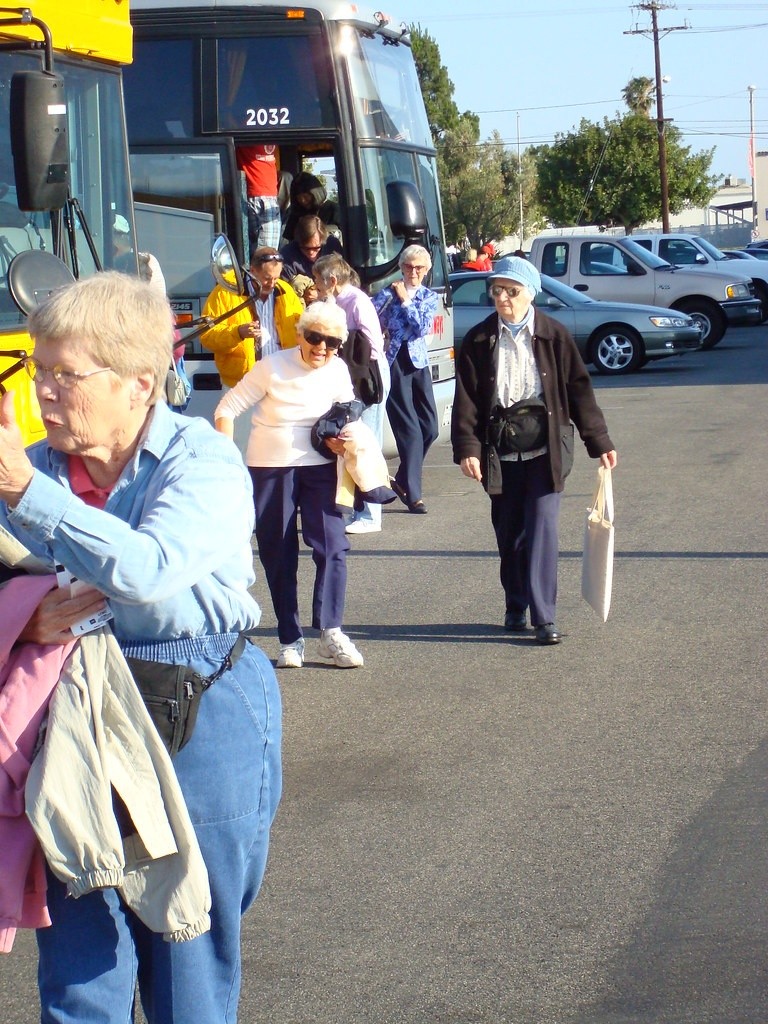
[275,637,305,667]
[317,626,363,667]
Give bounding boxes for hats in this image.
[486,256,543,295]
[480,246,490,254]
[486,244,496,255]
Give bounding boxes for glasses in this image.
[491,285,529,297]
[303,328,342,349]
[401,264,428,272]
[252,254,284,264]
[20,355,115,389]
[298,245,321,252]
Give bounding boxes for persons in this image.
[462,243,497,271]
[0,270,282,1024]
[373,245,440,513]
[111,236,192,414]
[235,141,282,255]
[279,214,392,533]
[213,301,364,670]
[450,256,616,644]
[282,172,341,242]
[515,250,527,259]
[198,247,305,464]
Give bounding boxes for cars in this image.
[554,238,768,324]
[448,270,704,377]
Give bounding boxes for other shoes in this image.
[390,480,407,505]
[408,502,427,514]
[344,517,381,533]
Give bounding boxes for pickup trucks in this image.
[529,232,764,352]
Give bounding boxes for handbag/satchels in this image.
[124,656,204,756]
[167,370,186,406]
[580,465,614,623]
[492,396,548,456]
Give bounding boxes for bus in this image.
[123,0,457,462]
[1,0,140,448]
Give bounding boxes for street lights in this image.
[748,85,758,243]
[654,73,672,235]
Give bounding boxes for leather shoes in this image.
[536,625,563,643]
[505,609,527,631]
